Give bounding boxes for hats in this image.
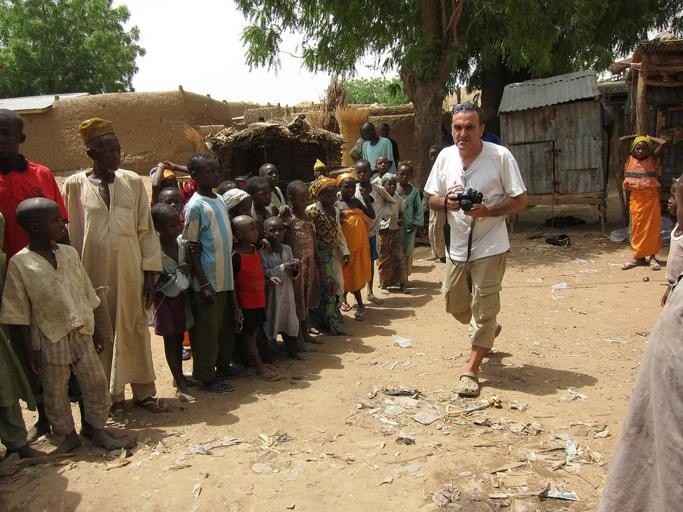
[336,172,358,187]
[312,176,336,198]
[160,169,176,183]
[313,158,326,172]
[78,118,115,145]
[222,188,251,210]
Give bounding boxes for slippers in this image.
[218,367,253,378]
[256,372,283,381]
[355,311,367,321]
[621,260,642,269]
[648,261,661,270]
[495,320,502,338]
[341,302,351,311]
[106,409,124,426]
[132,397,168,413]
[198,383,235,393]
[456,372,480,396]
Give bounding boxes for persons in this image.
[617,134,667,273]
[144,151,424,403]
[63,117,169,415]
[660,174,683,309]
[0,329,50,459]
[379,123,401,172]
[1,105,71,447]
[360,122,398,175]
[422,144,448,264]
[422,101,529,400]
[0,196,136,456]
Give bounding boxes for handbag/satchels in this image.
[312,238,332,264]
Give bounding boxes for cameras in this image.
[452,187,484,211]
